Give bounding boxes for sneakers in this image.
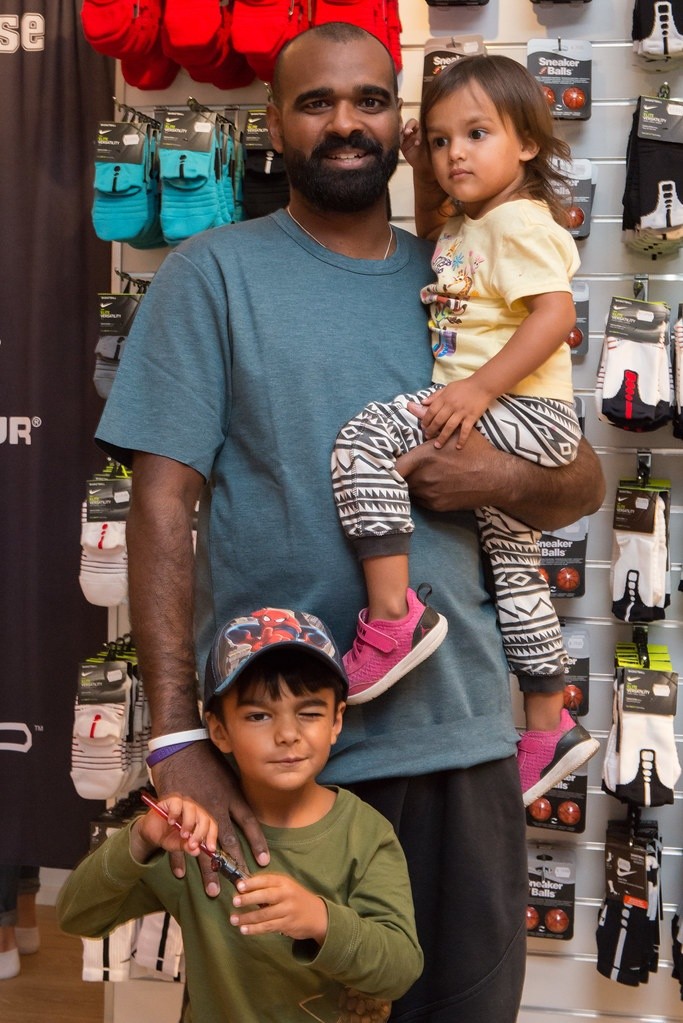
[520,707,600,809]
[342,593,450,705]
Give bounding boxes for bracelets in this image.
[145,726,206,768]
[148,728,210,752]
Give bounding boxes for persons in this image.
[329,52,602,809]
[0,865,42,982]
[92,20,607,899]
[54,606,425,1022]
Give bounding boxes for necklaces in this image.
[285,203,394,265]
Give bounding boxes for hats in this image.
[204,608,349,705]
